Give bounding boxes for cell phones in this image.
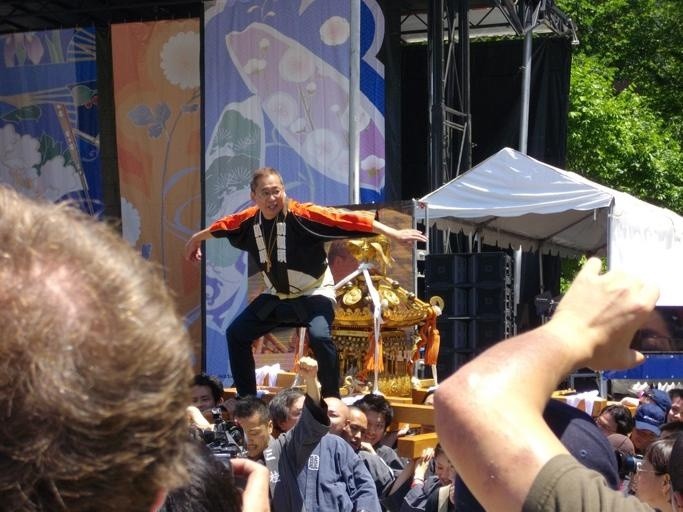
[630,306,683,355]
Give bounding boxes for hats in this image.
[635,403,665,436]
[454,400,622,512]
[606,434,634,456]
[642,389,672,413]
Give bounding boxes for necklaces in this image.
[261,210,287,274]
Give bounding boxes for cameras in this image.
[197,404,246,465]
[614,448,637,477]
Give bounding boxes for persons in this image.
[630,309,677,354]
[436,256,682,511]
[185,167,427,404]
[0,183,206,512]
[160,357,680,510]
